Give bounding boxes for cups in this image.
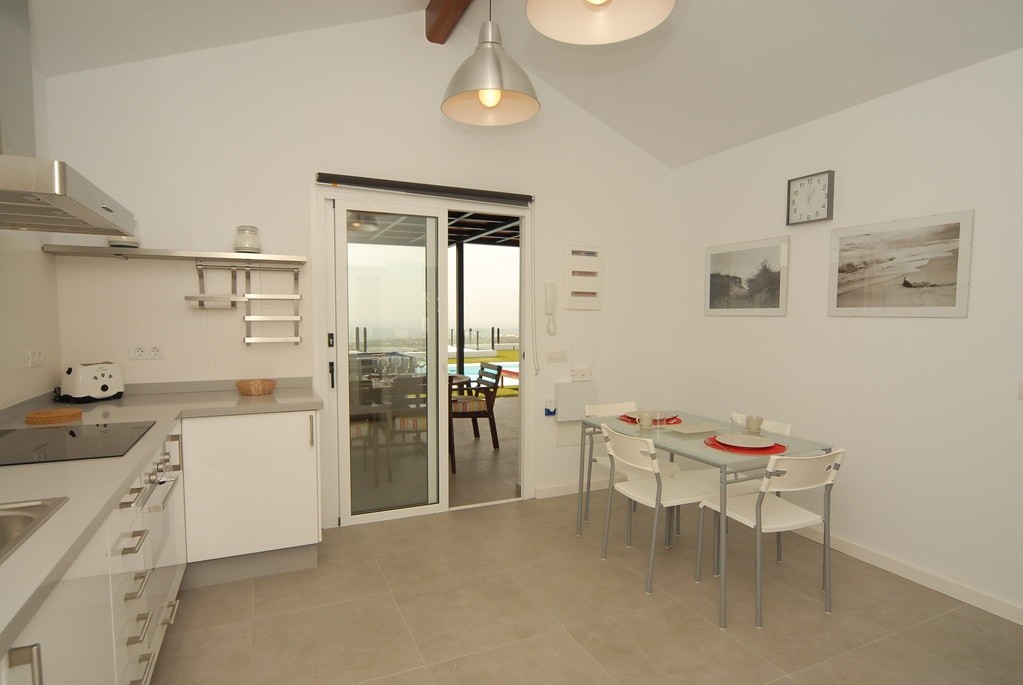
[745,416,763,433]
[636,406,667,429]
[731,415,747,435]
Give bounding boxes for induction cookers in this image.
[0,418,154,465]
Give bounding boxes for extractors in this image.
[0,0,136,237]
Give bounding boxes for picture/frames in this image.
[704,235,791,318]
[827,209,975,319]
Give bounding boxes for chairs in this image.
[583,399,637,524]
[693,449,847,630]
[600,412,793,596]
[350,363,502,488]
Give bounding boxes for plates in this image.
[716,434,776,448]
[626,411,679,419]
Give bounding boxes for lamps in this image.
[425,0,678,129]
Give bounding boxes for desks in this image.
[576,409,835,629]
[351,373,481,441]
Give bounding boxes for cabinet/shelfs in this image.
[0,408,323,685]
[41,244,306,345]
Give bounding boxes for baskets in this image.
[235,379,277,396]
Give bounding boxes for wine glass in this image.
[372,355,426,383]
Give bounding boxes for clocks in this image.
[786,170,834,226]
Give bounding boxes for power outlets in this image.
[128,343,165,360]
[27,348,45,368]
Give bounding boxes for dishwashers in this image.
[136,437,180,648]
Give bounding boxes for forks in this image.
[704,437,733,452]
